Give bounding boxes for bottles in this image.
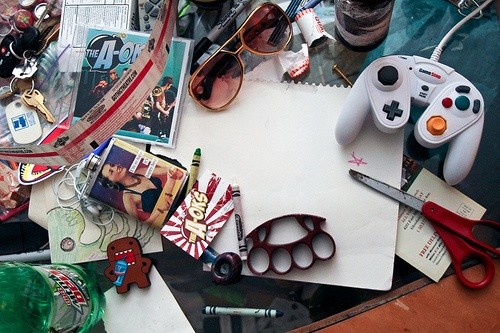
[335,0,395,52]
[0,262,106,333]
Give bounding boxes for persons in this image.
[97,163,177,224]
[106,68,120,91]
[156,76,173,134]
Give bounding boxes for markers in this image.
[192,0,252,63]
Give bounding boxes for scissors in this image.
[349,169,500,288]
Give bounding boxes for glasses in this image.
[187,1,295,112]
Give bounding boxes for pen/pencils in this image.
[0,249,50,263]
